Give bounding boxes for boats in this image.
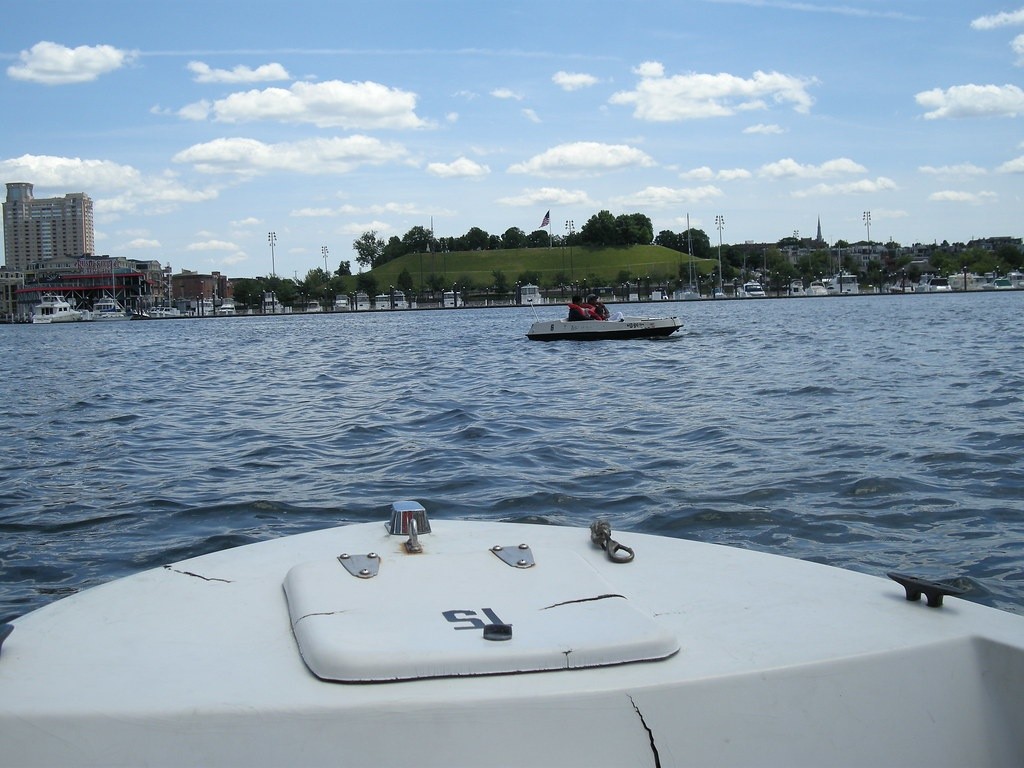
[147,307,180,318]
[525,317,684,341]
[982,279,1015,290]
[82,295,133,321]
[807,281,827,296]
[925,278,952,292]
[32,296,82,323]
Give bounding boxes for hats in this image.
[587,294,598,302]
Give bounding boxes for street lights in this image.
[201,292,204,316]
[879,270,882,293]
[322,246,330,273]
[637,278,640,302]
[863,211,871,261]
[793,230,800,266]
[839,270,842,293]
[515,280,521,305]
[197,296,199,316]
[452,282,457,307]
[571,282,574,300]
[390,284,395,309]
[272,291,275,313]
[441,289,444,306]
[622,283,624,301]
[964,266,967,290]
[212,293,215,316]
[775,272,779,297]
[788,276,790,295]
[301,292,304,312]
[566,220,574,282]
[715,215,725,294]
[902,267,906,292]
[249,294,252,309]
[268,232,277,278]
[993,265,1000,279]
[583,278,586,301]
[647,276,649,300]
[576,280,580,297]
[711,274,716,298]
[325,288,326,311]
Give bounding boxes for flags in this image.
[539,211,549,228]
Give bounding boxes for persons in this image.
[569,296,593,320]
[590,308,603,319]
[587,293,609,320]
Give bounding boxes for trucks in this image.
[738,284,766,297]
